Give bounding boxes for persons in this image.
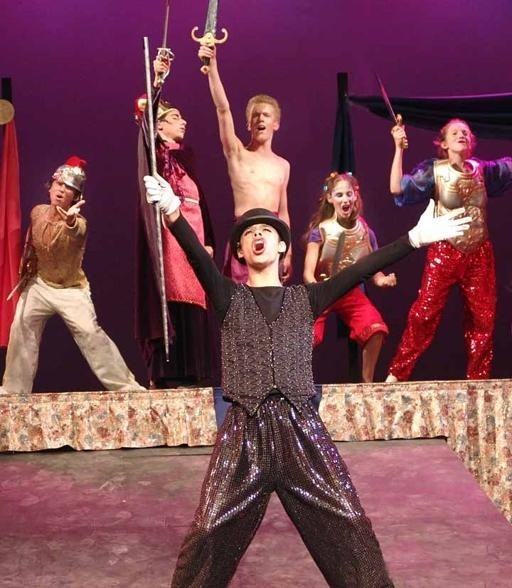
[135,54,214,389]
[143,171,474,588]
[197,44,293,286]
[386,118,512,384]
[302,171,398,385]
[2,156,146,395]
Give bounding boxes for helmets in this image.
[46,156,87,201]
[134,93,178,123]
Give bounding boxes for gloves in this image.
[144,171,181,217]
[408,198,473,249]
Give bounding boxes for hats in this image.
[230,208,291,265]
